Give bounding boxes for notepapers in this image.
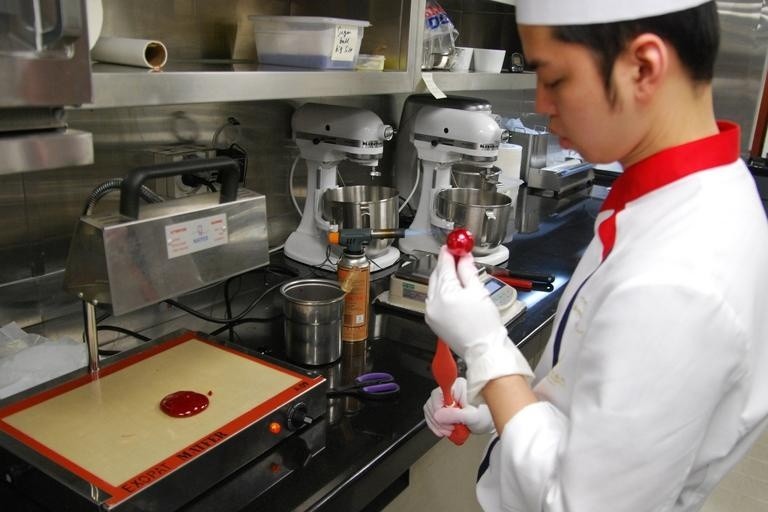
[422,72,449,103]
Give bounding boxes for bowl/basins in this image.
[450,46,472,72]
[322,186,400,253]
[474,47,506,73]
[434,187,512,256]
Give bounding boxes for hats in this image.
[512,1,709,27]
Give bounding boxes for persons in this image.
[422,0,768,512]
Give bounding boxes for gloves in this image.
[422,242,537,409]
[422,376,498,440]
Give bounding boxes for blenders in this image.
[283,103,415,285]
[400,106,514,275]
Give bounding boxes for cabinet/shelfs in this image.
[64,0,417,110]
[414,1,540,92]
